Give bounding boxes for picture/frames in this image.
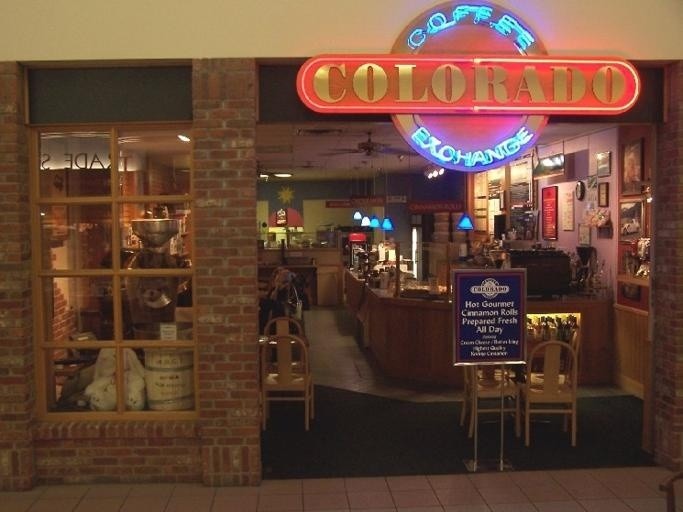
[596,180,610,208]
[618,197,646,246]
[619,135,646,197]
[595,151,613,179]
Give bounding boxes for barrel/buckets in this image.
[142,346,197,410]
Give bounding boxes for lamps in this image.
[346,151,398,233]
[452,172,476,233]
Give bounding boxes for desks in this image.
[254,330,311,349]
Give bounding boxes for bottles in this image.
[124,218,181,343]
[527,315,582,344]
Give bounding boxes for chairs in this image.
[259,316,310,371]
[459,350,522,440]
[256,333,318,433]
[518,338,581,448]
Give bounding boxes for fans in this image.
[319,129,421,159]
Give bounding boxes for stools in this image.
[476,367,517,380]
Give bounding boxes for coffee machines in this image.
[488,250,508,269]
[574,242,600,292]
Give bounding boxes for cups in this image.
[379,272,388,288]
[428,276,440,294]
